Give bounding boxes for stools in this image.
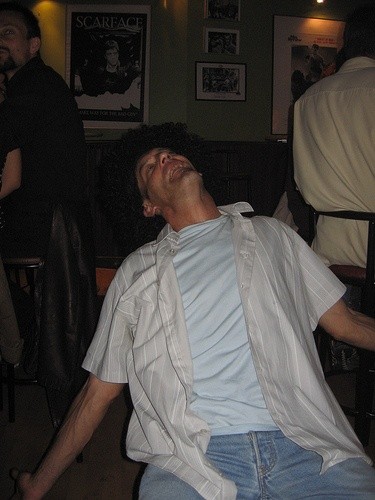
[0,255,56,430]
[298,206,375,451]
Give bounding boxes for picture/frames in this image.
[270,14,347,136]
[203,0,241,22]
[202,27,241,56]
[194,61,247,102]
[64,3,152,130]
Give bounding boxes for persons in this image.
[290,69,314,101]
[266,42,375,382]
[208,31,237,56]
[70,38,133,92]
[303,44,328,82]
[202,67,239,93]
[9,146,375,500]
[0,0,84,382]
